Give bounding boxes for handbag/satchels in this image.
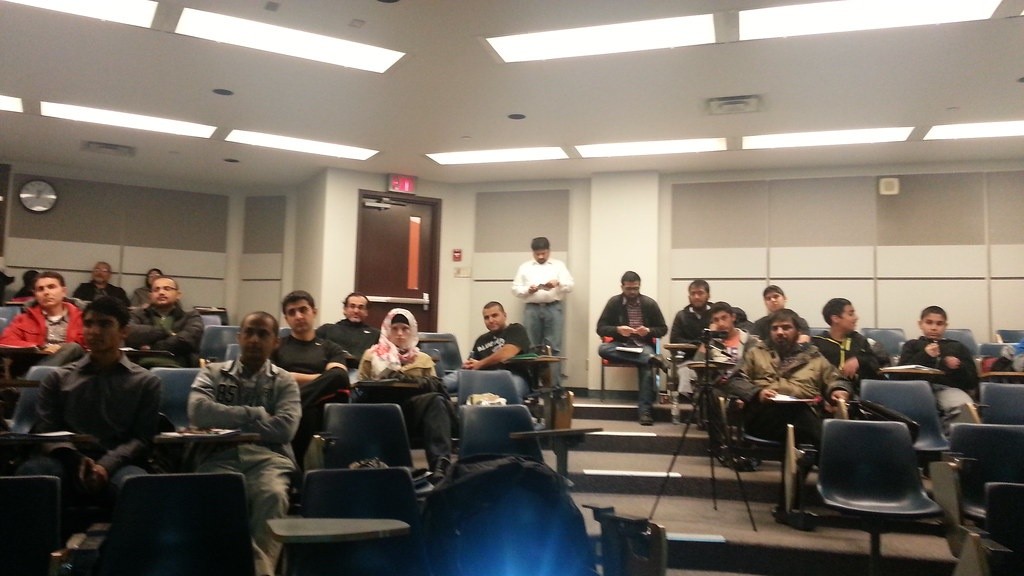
[849,399,921,444]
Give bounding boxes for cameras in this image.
[702,328,729,342]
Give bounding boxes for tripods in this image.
[648,342,757,532]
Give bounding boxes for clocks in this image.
[17,179,60,214]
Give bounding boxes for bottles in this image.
[671,402,680,425]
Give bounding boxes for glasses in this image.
[153,286,178,291]
[94,267,110,272]
[147,273,160,278]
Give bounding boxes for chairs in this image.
[224,343,243,363]
[947,422,1024,535]
[454,367,523,405]
[301,466,434,576]
[0,474,70,576]
[728,395,802,470]
[455,401,546,466]
[318,399,415,468]
[594,319,663,401]
[809,327,830,336]
[0,305,24,342]
[978,382,1024,425]
[861,327,910,355]
[97,470,253,576]
[199,321,241,361]
[422,452,593,576]
[202,315,222,325]
[420,348,445,377]
[979,342,1024,360]
[859,377,953,469]
[982,482,1024,576]
[281,327,296,339]
[813,416,947,576]
[942,327,978,357]
[996,328,1024,343]
[148,364,200,427]
[418,332,462,369]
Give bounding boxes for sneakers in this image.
[637,399,654,426]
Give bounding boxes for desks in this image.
[980,368,1023,380]
[265,514,411,544]
[506,420,603,478]
[154,419,262,463]
[879,365,946,378]
[358,372,429,394]
[687,360,737,429]
[508,358,562,363]
[663,342,700,390]
[0,429,99,442]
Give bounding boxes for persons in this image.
[186,290,531,576]
[0,261,205,576]
[1011,337,1024,372]
[898,306,982,433]
[512,237,576,399]
[670,279,889,528]
[596,271,670,426]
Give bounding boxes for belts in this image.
[525,300,561,306]
